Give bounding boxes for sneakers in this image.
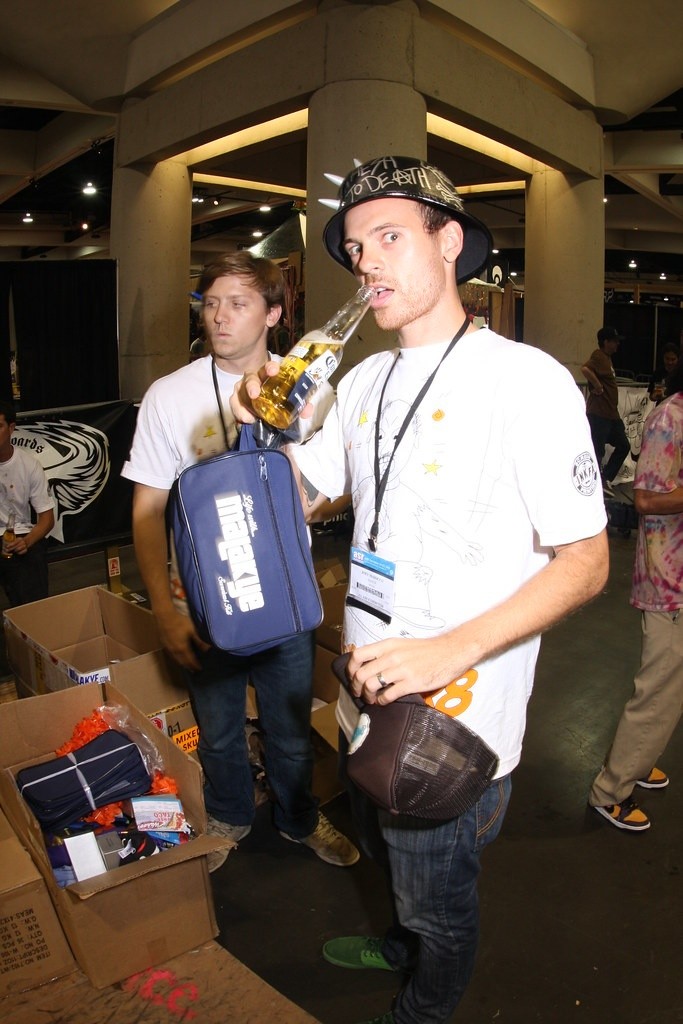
[601,478,614,497]
[595,796,650,832]
[636,769,670,790]
[322,935,411,975]
[200,815,253,872]
[280,811,360,868]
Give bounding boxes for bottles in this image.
[2,513,17,560]
[251,284,376,430]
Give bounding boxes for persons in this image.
[587,359,683,834]
[580,327,631,498]
[119,250,368,876]
[264,155,612,1024]
[647,342,683,408]
[0,403,56,677]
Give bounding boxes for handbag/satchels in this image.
[172,428,329,655]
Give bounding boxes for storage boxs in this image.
[0,557,348,997]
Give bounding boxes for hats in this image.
[597,326,623,341]
[330,651,502,823]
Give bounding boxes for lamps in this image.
[82,182,96,195]
[252,228,263,237]
[659,273,667,280]
[22,213,34,223]
[214,197,221,205]
[259,195,273,212]
[628,260,637,268]
